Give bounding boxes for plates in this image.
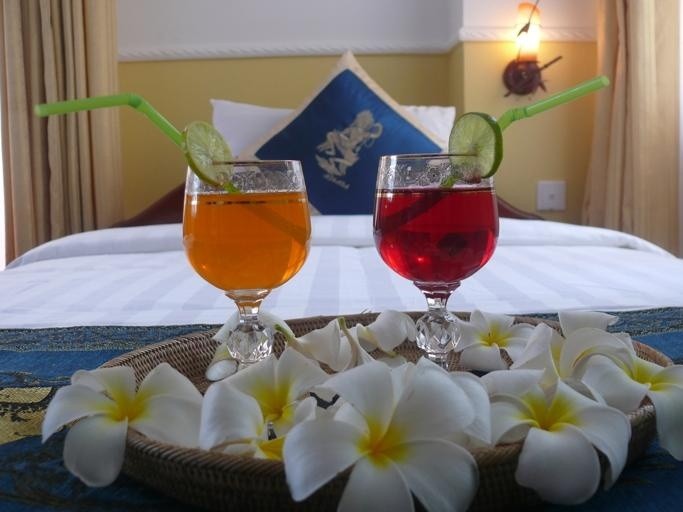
[65,309,678,512]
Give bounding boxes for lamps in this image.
[502,0,563,98]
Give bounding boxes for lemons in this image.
[447,113,504,179]
[182,118,234,186]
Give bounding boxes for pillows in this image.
[208,98,455,163]
[238,48,449,213]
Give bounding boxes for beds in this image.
[0,175,683,511]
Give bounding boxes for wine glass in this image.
[371,153,501,375]
[182,160,311,373]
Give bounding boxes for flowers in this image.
[42,308,683,512]
